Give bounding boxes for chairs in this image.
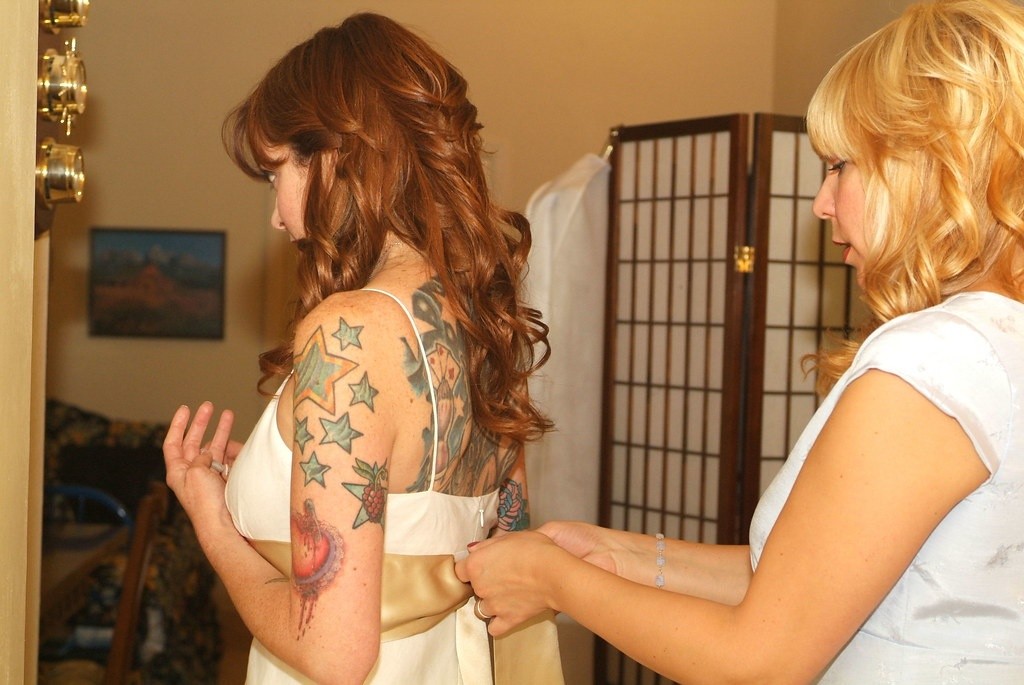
[39,480,169,684]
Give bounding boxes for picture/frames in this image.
[87,226,225,338]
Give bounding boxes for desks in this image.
[42,519,130,612]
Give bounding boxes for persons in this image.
[454,3,1024,685]
[162,11,556,685]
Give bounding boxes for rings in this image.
[474,599,492,621]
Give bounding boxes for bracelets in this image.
[655,533,666,590]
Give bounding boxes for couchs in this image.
[40,396,224,684]
